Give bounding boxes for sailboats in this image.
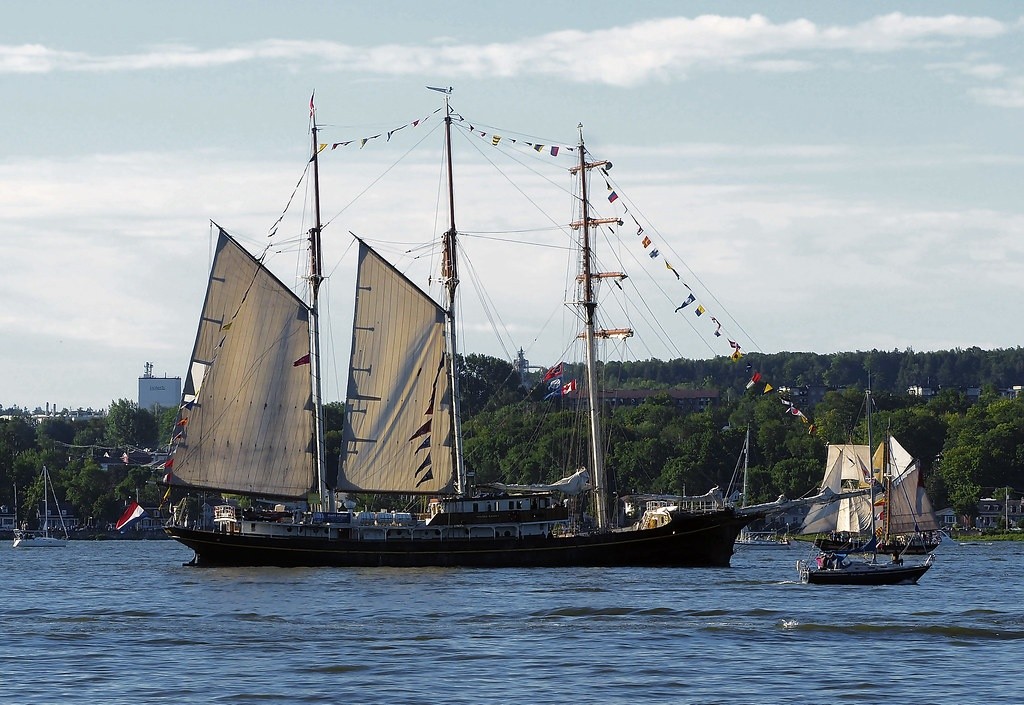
[139,81,886,572]
[722,422,790,546]
[792,367,941,585]
[11,464,70,548]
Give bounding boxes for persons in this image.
[816,552,837,572]
[828,530,938,546]
[891,555,903,566]
[760,533,787,541]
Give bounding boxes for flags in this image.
[544,362,562,381]
[544,379,576,401]
[116,501,149,534]
[164,92,815,491]
[548,377,562,390]
[874,498,885,506]
[875,526,882,535]
[874,511,883,520]
